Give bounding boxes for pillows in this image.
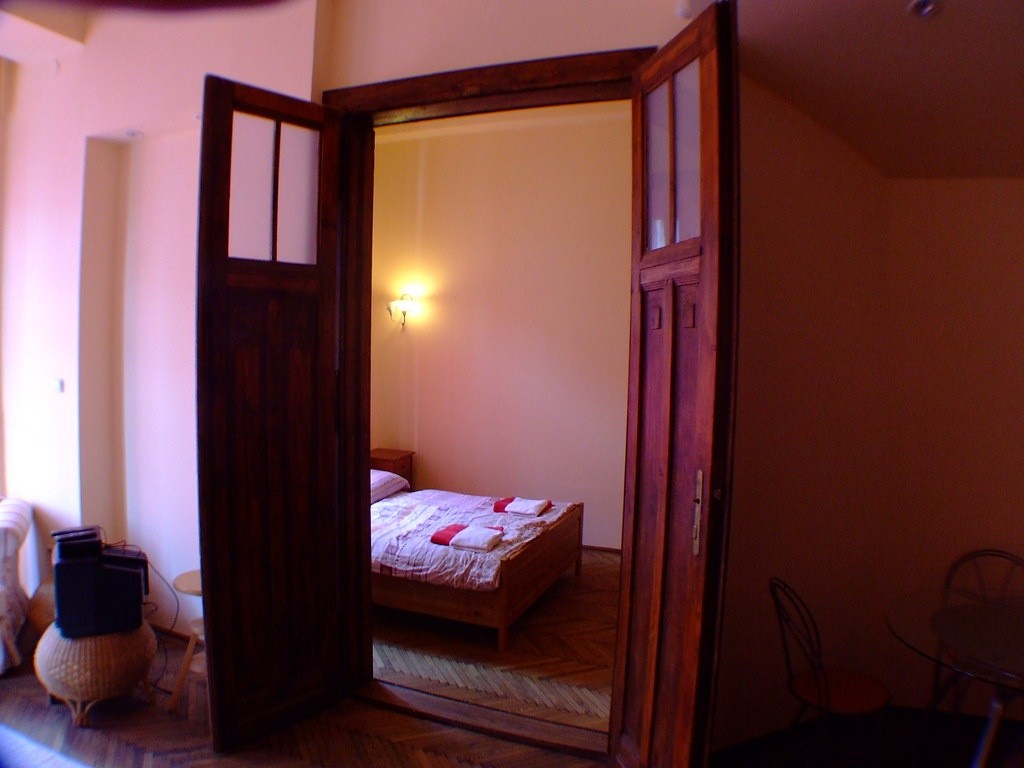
[371,469,410,505]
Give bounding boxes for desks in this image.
[884,590,1024,767]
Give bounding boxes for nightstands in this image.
[369,448,415,492]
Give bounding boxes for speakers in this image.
[49,525,150,641]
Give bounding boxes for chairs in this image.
[930,548,1023,746]
[764,577,936,768]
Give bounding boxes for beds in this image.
[370,489,584,653]
